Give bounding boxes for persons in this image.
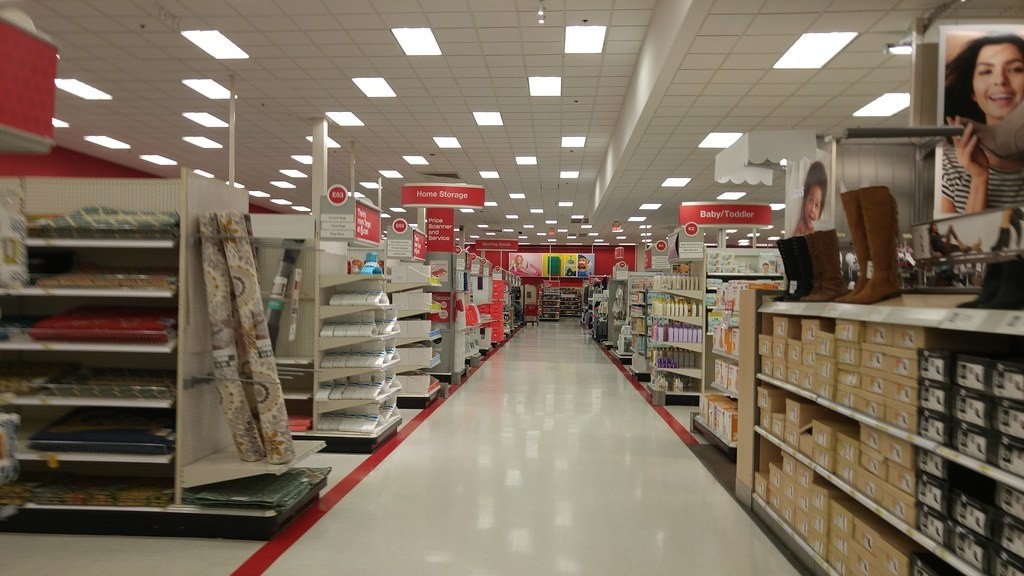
[762,262,770,272]
[792,159,827,238]
[940,32,1024,218]
[927,207,1024,262]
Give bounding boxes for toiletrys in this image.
[651,297,702,317]
[653,347,695,367]
[651,323,703,342]
[653,274,699,290]
[650,371,694,392]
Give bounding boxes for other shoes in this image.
[960,242,968,251]
[973,236,983,253]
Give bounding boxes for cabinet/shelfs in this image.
[1,166,331,542]
[647,245,708,399]
[387,259,441,399]
[588,294,604,338]
[560,288,582,316]
[750,288,1024,576]
[538,292,560,322]
[707,273,783,397]
[315,221,402,438]
[691,246,784,452]
[616,275,646,355]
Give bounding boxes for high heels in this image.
[1009,207,1024,245]
[991,226,1010,252]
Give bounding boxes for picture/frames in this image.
[933,25,1024,221]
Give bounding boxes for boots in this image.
[959,99,1024,157]
[834,190,874,302]
[804,228,848,302]
[955,262,1001,308]
[800,231,825,301]
[978,260,1024,310]
[784,236,814,301]
[772,239,790,302]
[845,186,902,305]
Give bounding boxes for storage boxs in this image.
[711,326,738,354]
[699,394,741,444]
[391,266,432,282]
[392,292,433,311]
[396,346,434,365]
[395,375,431,393]
[398,320,431,339]
[754,316,1024,576]
[714,360,740,391]
[716,281,781,310]
